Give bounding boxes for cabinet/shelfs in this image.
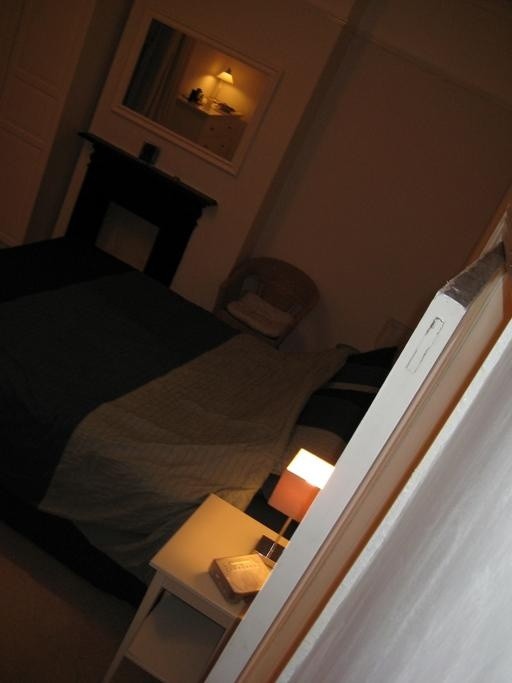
[0,1,132,249]
[102,493,285,683]
[171,96,243,159]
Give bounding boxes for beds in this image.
[0,239,397,608]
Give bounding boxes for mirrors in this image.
[111,7,284,177]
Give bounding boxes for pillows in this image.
[323,347,398,407]
[258,396,365,517]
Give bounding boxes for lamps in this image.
[251,448,335,570]
[212,67,233,101]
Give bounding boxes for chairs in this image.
[212,256,319,350]
